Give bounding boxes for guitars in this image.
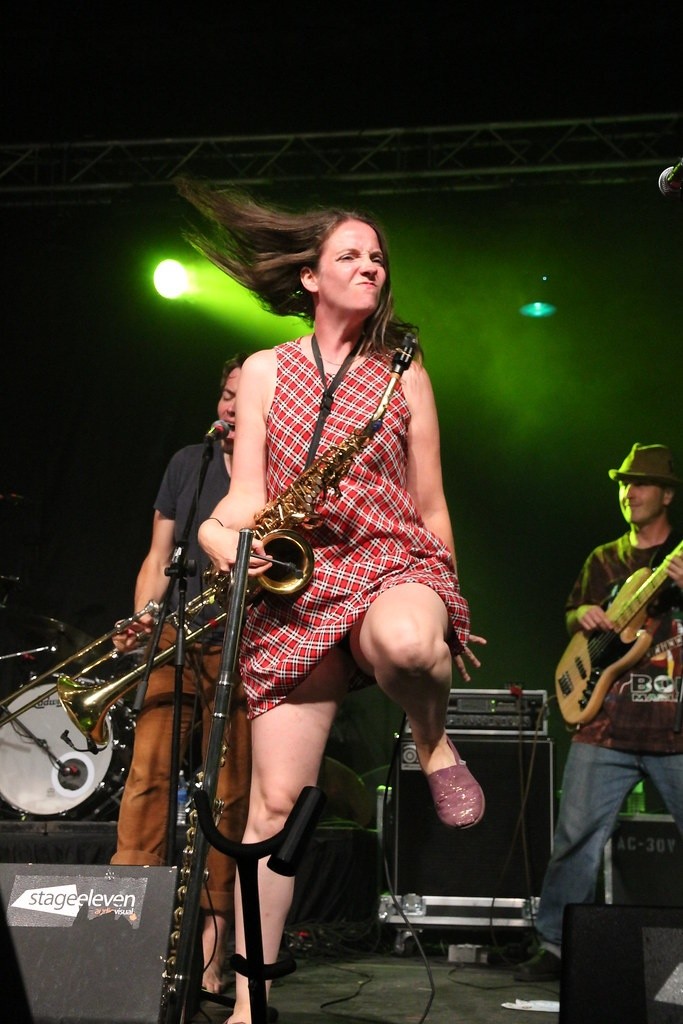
[554,540,683,724]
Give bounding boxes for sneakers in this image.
[514,949,562,981]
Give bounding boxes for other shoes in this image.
[415,735,485,830]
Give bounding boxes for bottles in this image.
[379,891,427,954]
[176,770,187,825]
[627,779,646,813]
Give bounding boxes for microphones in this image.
[205,420,229,442]
[658,159,683,197]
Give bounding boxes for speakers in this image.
[394,734,554,898]
[559,903,682,1024]
[0,864,203,1024]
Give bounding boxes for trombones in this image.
[0,585,252,727]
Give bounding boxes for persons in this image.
[512,443,683,982]
[112,351,252,992]
[179,183,487,1024]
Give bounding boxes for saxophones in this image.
[204,330,419,612]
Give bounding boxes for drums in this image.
[0,673,138,822]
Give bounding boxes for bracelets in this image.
[208,518,223,526]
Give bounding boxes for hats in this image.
[607,443,683,486]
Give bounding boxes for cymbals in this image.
[0,610,106,681]
